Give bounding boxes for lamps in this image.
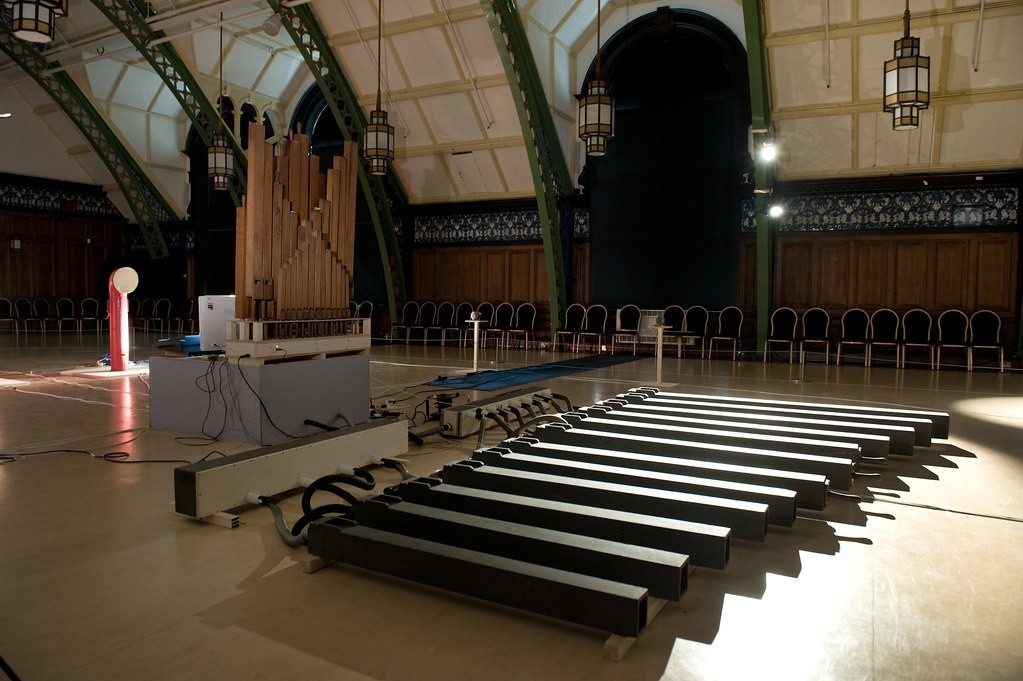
[573,0,615,156]
[4,0,68,43]
[882,0,931,132]
[364,0,395,175]
[208,12,235,190]
[263,13,282,36]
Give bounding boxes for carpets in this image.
[425,348,656,390]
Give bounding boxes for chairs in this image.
[0,298,1003,372]
